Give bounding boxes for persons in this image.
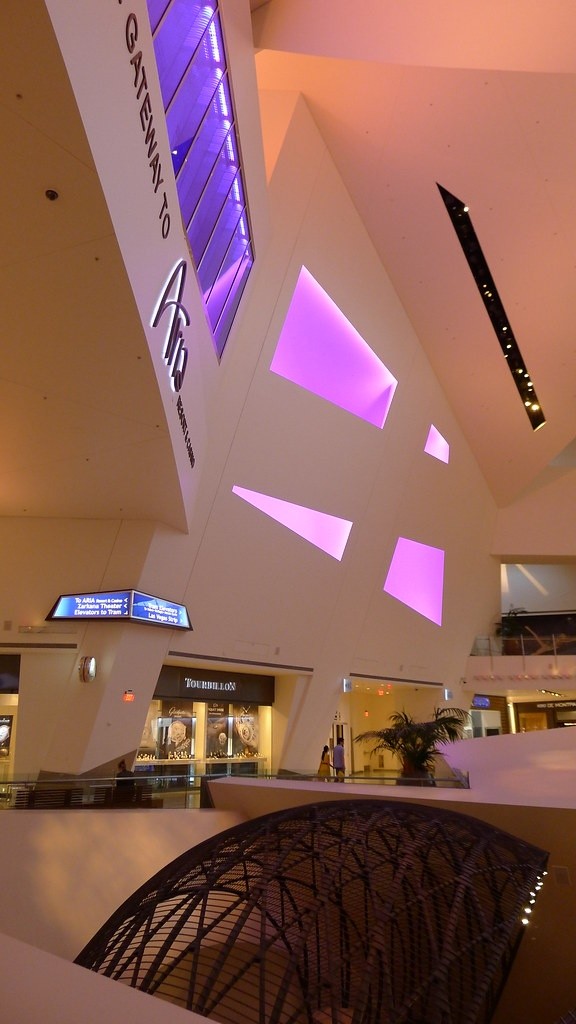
[333,737,345,783]
[319,744,332,783]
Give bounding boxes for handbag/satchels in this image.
[337,771,345,782]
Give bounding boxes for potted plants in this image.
[350,704,477,788]
[493,607,535,655]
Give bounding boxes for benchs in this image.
[3,784,163,809]
